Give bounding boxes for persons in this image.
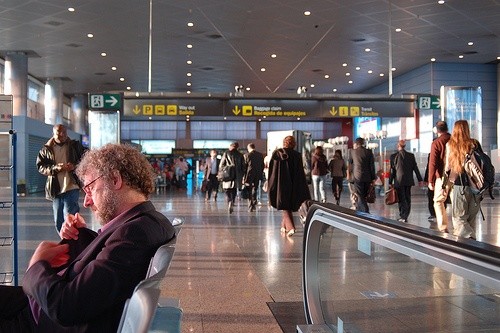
[38,122,89,240]
[440,120,493,252]
[267,136,310,237]
[387,139,423,225]
[425,137,446,220]
[202,134,377,219]
[426,122,463,236]
[169,155,196,190]
[0,142,179,333]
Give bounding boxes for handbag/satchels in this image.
[222,152,236,182]
[384,185,398,205]
[366,183,376,203]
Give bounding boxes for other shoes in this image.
[281,227,286,233]
[428,215,436,219]
[397,218,407,223]
[286,229,295,236]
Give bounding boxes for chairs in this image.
[116,216,186,333]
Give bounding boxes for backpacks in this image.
[459,138,495,200]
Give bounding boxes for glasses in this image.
[82,175,103,196]
